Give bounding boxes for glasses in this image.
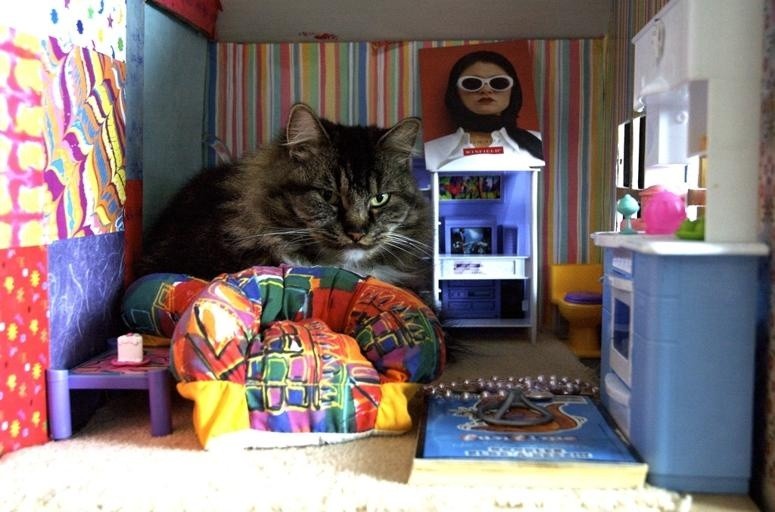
[458,76,514,92]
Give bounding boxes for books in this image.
[405,386,650,490]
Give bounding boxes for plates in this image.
[110,358,154,367]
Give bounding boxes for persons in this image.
[439,176,500,256]
[424,49,546,172]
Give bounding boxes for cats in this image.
[132,102,449,325]
